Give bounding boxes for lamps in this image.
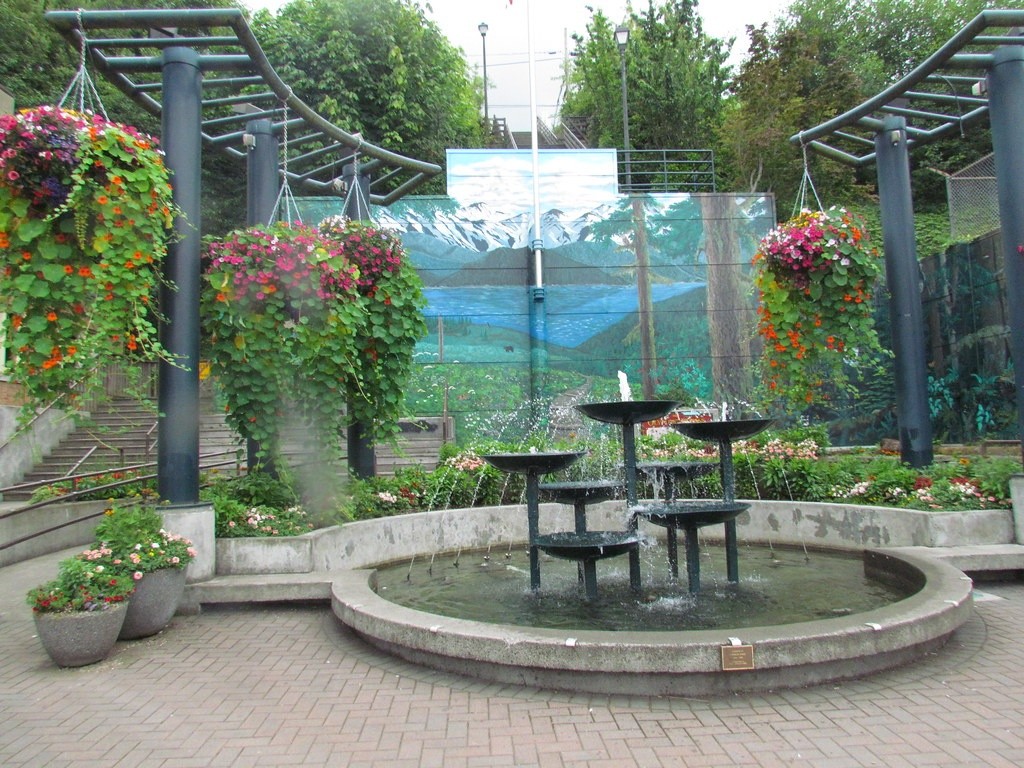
[891,130,901,146]
[971,79,986,95]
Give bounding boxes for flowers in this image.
[28,560,124,609]
[743,205,894,400]
[203,211,422,458]
[0,70,181,450]
[77,507,197,573]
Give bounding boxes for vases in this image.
[124,562,192,638]
[32,603,126,667]
